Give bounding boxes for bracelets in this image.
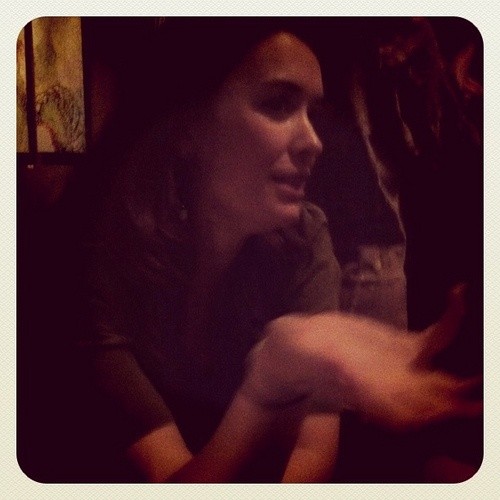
[237,380,313,411]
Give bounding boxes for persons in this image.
[307,14,480,334]
[15,15,484,484]
